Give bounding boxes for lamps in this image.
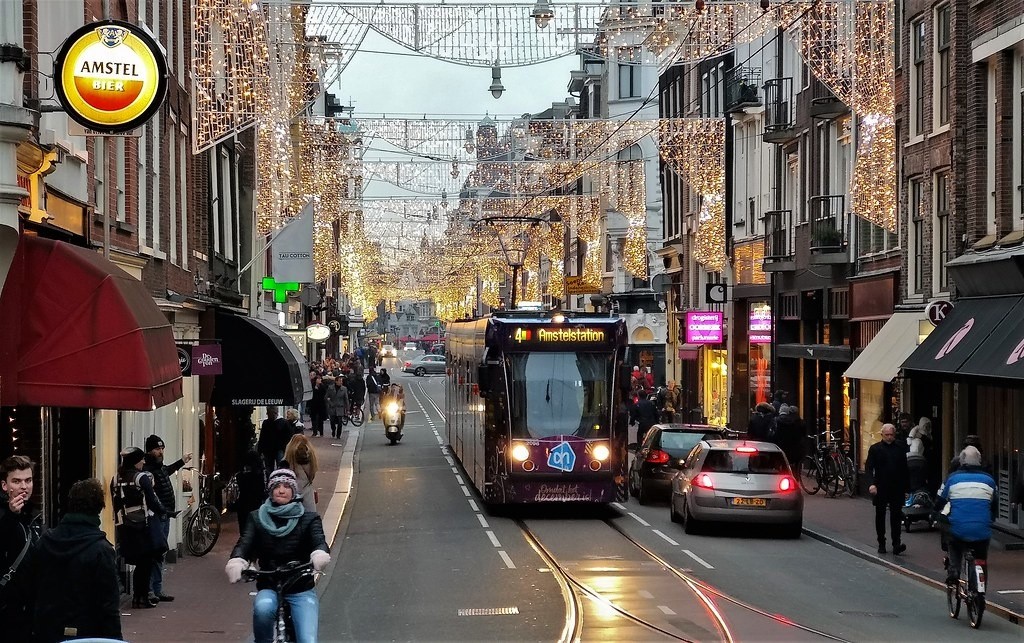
[439,188,449,209]
[487,60,506,99]
[432,205,439,220]
[479,114,498,138]
[527,0,555,28]
[426,210,432,224]
[449,156,460,179]
[462,123,476,154]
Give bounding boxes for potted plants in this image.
[811,220,840,253]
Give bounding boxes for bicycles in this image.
[341,391,365,427]
[240,560,327,643]
[799,429,857,500]
[933,505,988,629]
[181,465,224,556]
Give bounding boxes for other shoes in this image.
[131,599,157,608]
[332,427,336,437]
[371,416,375,420]
[312,433,318,437]
[893,544,906,554]
[878,542,887,554]
[319,431,323,436]
[148,592,159,603]
[156,591,178,601]
[337,434,341,439]
[946,570,959,584]
[378,412,382,418]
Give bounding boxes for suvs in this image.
[627,423,729,506]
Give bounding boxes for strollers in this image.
[902,452,941,533]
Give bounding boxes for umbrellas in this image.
[394,333,446,351]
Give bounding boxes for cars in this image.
[400,354,447,377]
[666,439,804,539]
[379,345,399,358]
[402,342,418,352]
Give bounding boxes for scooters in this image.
[380,393,406,445]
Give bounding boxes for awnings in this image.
[843,313,929,382]
[899,297,1024,389]
[199,314,313,405]
[0,232,184,412]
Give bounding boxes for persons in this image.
[366,366,383,421]
[747,390,810,481]
[629,366,681,454]
[306,357,366,439]
[377,349,383,367]
[372,338,379,346]
[342,344,377,369]
[110,434,193,609]
[379,368,390,394]
[25,479,123,643]
[225,468,331,643]
[0,456,43,643]
[236,405,318,536]
[865,413,998,616]
[422,342,445,355]
[379,382,406,434]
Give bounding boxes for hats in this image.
[959,445,982,466]
[778,404,790,416]
[120,447,146,468]
[267,468,298,501]
[145,435,165,452]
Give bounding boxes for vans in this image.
[430,344,445,356]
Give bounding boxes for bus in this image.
[444,308,634,514]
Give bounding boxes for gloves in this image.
[309,550,331,570]
[225,557,249,583]
